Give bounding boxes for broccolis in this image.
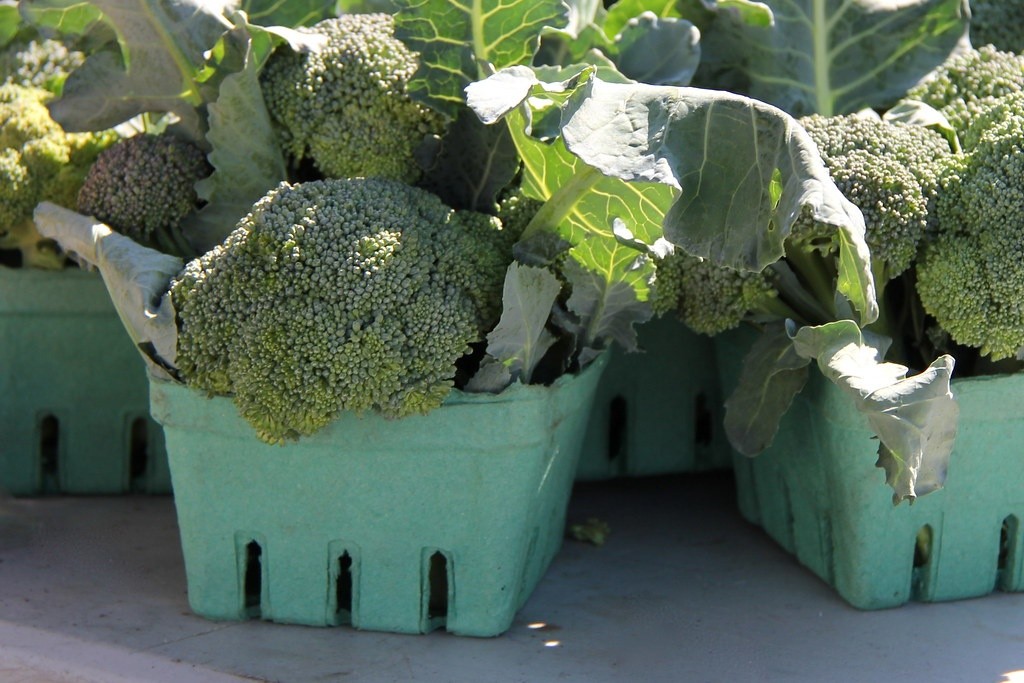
[0,0,1024,506]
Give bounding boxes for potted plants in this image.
[575,0,767,483]
[718,0,1024,609]
[0,2,259,496]
[32,0,875,639]
